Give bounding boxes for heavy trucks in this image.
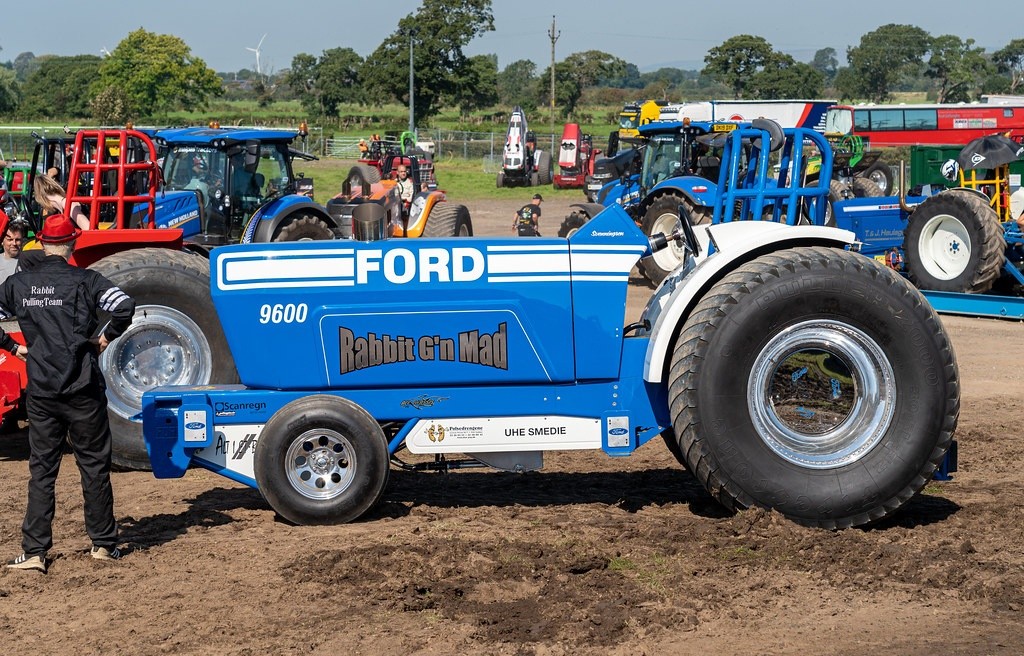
[650,99,839,146]
[619,100,674,147]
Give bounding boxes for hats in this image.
[533,194,544,202]
[36,214,82,243]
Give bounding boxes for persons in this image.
[34,167,90,230]
[1018,209,1024,253]
[0,148,7,188]
[358,139,368,159]
[581,140,590,173]
[0,222,28,335]
[0,214,135,574]
[193,152,208,175]
[512,194,544,237]
[369,134,379,142]
[420,182,428,192]
[394,165,413,215]
[979,163,1013,222]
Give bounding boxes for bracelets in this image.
[11,343,19,355]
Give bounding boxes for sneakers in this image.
[90,545,127,560]
[6,553,46,572]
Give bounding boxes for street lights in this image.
[400,25,421,130]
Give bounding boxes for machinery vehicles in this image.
[554,124,602,188]
[129,120,961,528]
[326,154,473,238]
[497,107,554,188]
[358,129,436,183]
[0,121,345,249]
[556,119,1024,321]
[0,127,242,473]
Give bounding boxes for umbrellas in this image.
[959,135,1024,171]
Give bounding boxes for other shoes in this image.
[0,447,25,460]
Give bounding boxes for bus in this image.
[824,103,1024,146]
